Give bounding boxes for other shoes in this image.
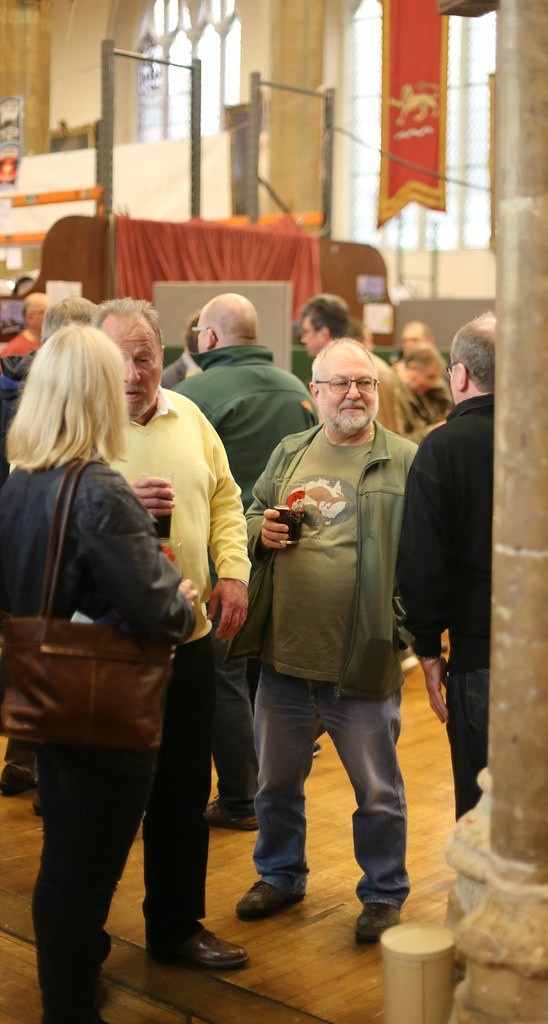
[0,775,39,796]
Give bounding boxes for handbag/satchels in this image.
[0,459,175,754]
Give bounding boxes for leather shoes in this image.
[150,927,249,969]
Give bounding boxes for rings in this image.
[192,601,194,607]
[191,591,197,597]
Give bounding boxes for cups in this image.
[272,479,306,544]
[142,473,174,539]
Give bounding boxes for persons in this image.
[0,322,200,1024]
[0,292,51,356]
[160,313,203,388]
[300,296,419,677]
[224,338,419,940]
[93,298,251,968]
[0,296,98,818]
[164,293,319,830]
[349,319,455,435]
[392,309,496,814]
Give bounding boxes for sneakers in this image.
[203,795,259,831]
[355,901,402,943]
[235,880,308,920]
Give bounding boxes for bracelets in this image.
[416,656,437,662]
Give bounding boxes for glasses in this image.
[446,361,469,378]
[315,377,378,394]
[191,326,219,342]
